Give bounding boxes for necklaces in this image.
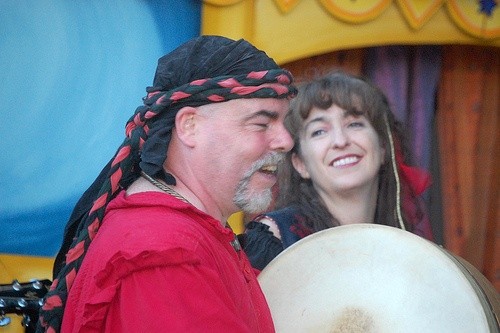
[140,170,241,252]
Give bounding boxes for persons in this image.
[235,70,435,271]
[37,35,298,333]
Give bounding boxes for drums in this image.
[257,224,500,333]
